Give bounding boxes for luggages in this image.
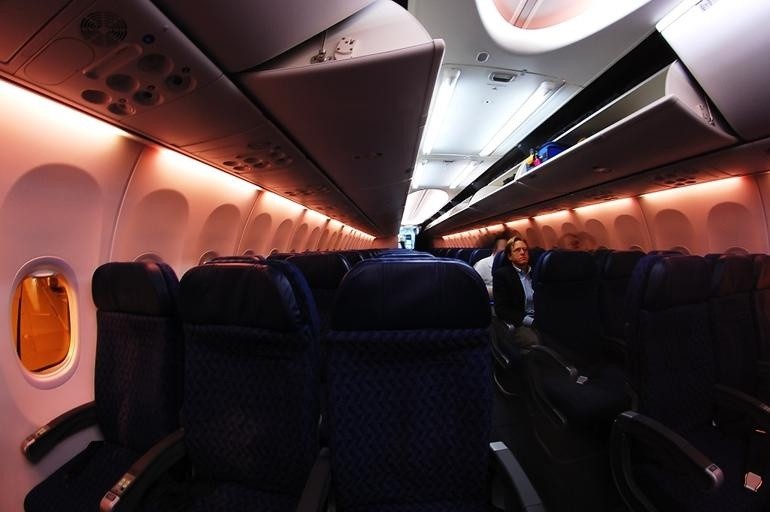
[526,140,568,170]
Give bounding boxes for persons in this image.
[495,236,542,351]
[470,235,510,318]
